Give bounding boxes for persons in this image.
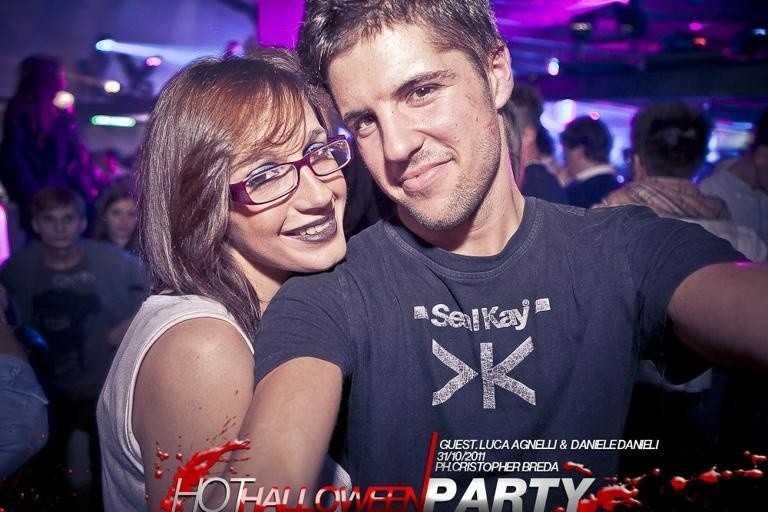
[0,47,104,240]
[1,184,155,510]
[584,96,742,465]
[89,178,146,256]
[101,148,128,185]
[697,105,768,250]
[497,85,571,208]
[99,41,357,512]
[0,286,52,484]
[557,113,625,210]
[198,0,768,511]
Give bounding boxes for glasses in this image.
[228,134,354,207]
